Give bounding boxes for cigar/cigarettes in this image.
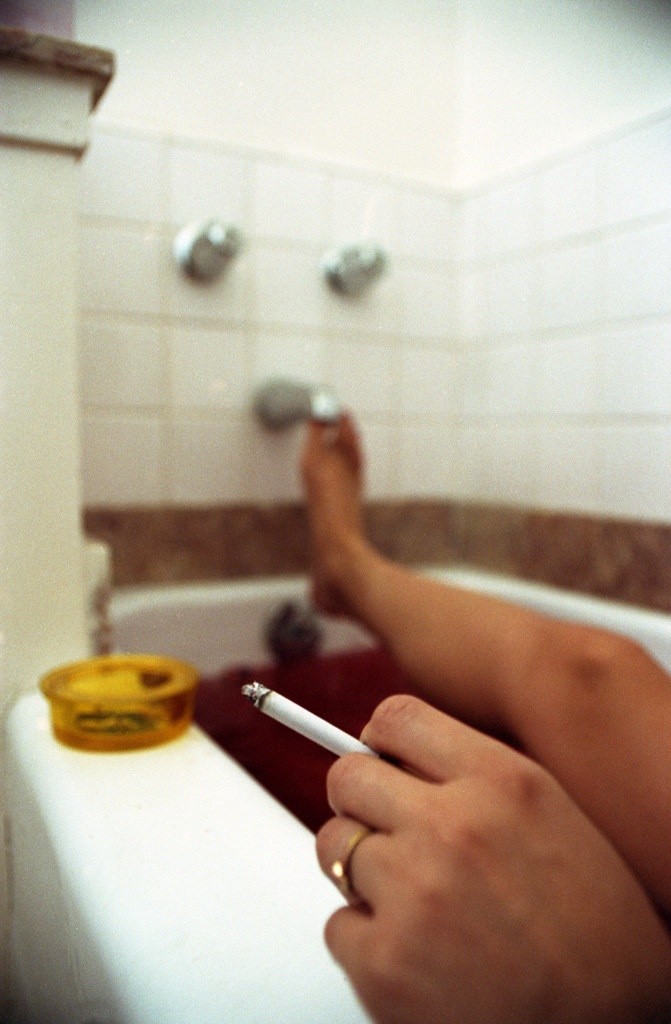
[241,681,381,758]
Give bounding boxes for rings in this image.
[334,828,371,897]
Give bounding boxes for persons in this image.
[300,412,671,1023]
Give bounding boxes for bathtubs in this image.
[0,556,671,1024]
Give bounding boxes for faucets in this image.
[257,374,342,440]
